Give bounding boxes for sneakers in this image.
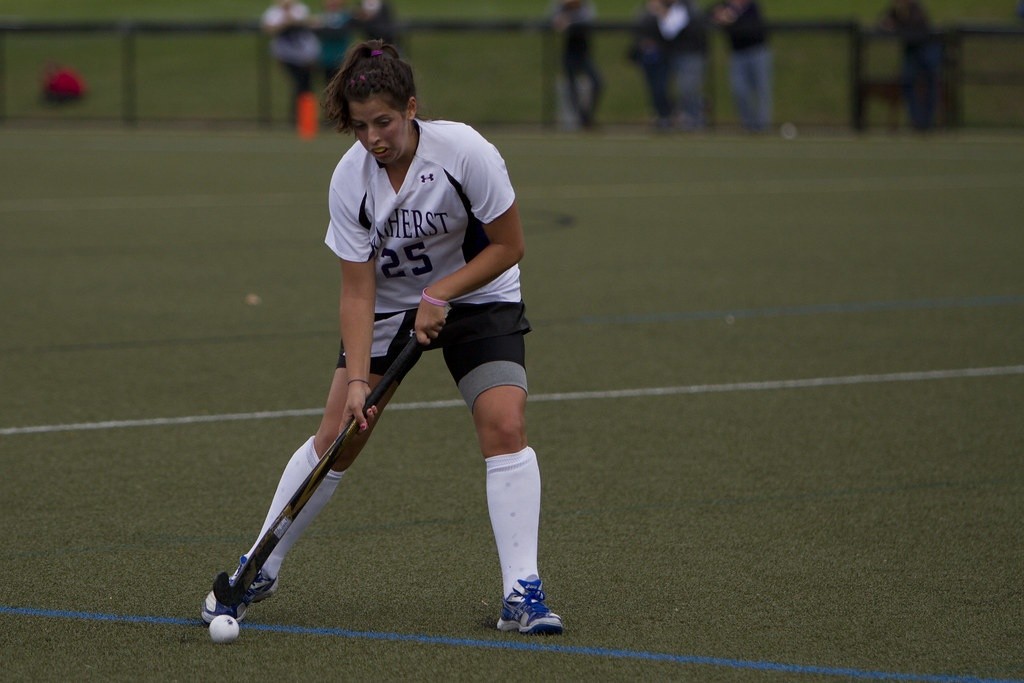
[201,553,277,625]
[494,576,564,637]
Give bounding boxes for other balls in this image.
[208,614,240,645]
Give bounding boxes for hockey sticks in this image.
[210,335,418,609]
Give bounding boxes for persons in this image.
[548,1,609,134]
[199,37,568,639]
[628,0,778,136]
[261,1,406,140]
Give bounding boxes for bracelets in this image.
[421,288,448,307]
[347,379,371,388]
